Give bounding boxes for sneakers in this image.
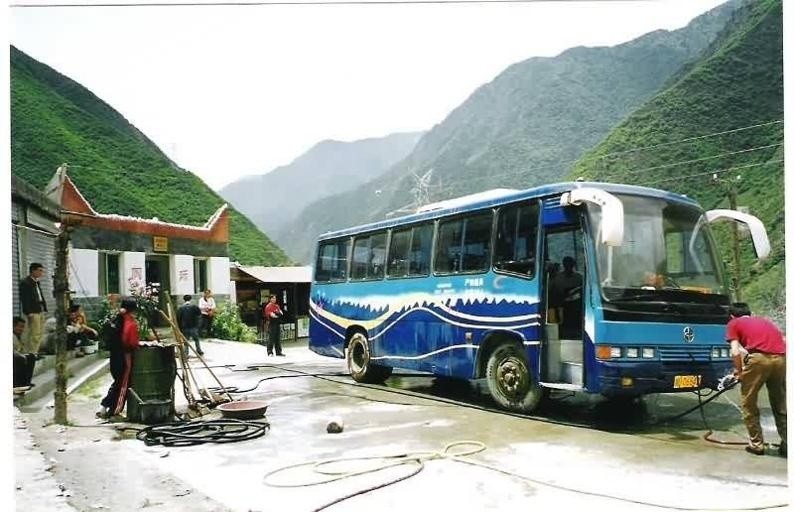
[95,412,129,422]
[268,352,285,357]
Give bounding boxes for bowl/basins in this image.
[215,400,270,419]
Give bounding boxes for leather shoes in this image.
[778,443,787,457]
[35,354,46,360]
[746,447,763,455]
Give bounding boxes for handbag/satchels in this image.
[104,328,123,349]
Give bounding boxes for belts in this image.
[748,350,760,353]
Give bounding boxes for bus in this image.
[307,178,772,413]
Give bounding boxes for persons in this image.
[724,301,786,460]
[264,295,286,357]
[176,294,204,360]
[197,288,216,338]
[551,257,584,339]
[13,315,35,392]
[95,298,139,422]
[42,303,98,357]
[20,262,49,360]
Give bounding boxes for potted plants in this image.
[80,338,96,354]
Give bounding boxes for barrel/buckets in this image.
[123,341,175,423]
